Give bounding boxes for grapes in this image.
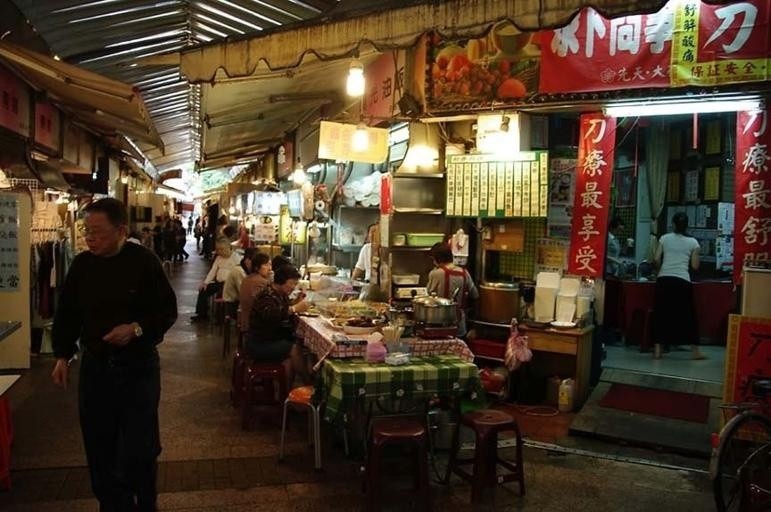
[439,59,511,96]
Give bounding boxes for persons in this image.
[128,214,316,385]
[352,223,376,282]
[427,244,479,343]
[51,196,179,511]
[607,215,635,275]
[649,212,706,359]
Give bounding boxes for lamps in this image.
[286,127,310,188]
[277,145,287,166]
[349,81,373,154]
[344,49,370,102]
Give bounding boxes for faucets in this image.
[636,258,649,282]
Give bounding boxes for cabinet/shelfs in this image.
[1,319,23,396]
[378,170,460,315]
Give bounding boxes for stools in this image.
[362,408,526,504]
[0,393,24,489]
[208,265,330,472]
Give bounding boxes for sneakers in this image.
[651,343,709,360]
[190,313,208,321]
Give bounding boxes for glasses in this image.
[78,224,121,238]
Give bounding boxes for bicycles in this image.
[706,378,770,512]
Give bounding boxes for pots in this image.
[480,275,536,322]
[412,288,461,327]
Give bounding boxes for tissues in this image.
[365,329,388,362]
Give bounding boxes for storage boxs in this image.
[406,233,446,247]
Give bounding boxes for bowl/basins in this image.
[343,324,378,335]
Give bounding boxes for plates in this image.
[551,321,576,328]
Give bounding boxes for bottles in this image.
[557,376,574,411]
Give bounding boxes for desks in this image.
[607,273,739,348]
[515,320,596,410]
[285,288,481,468]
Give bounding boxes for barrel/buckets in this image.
[558,378,576,412]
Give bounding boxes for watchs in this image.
[130,320,145,340]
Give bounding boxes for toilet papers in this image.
[315,201,339,226]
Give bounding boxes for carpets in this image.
[597,382,711,425]
